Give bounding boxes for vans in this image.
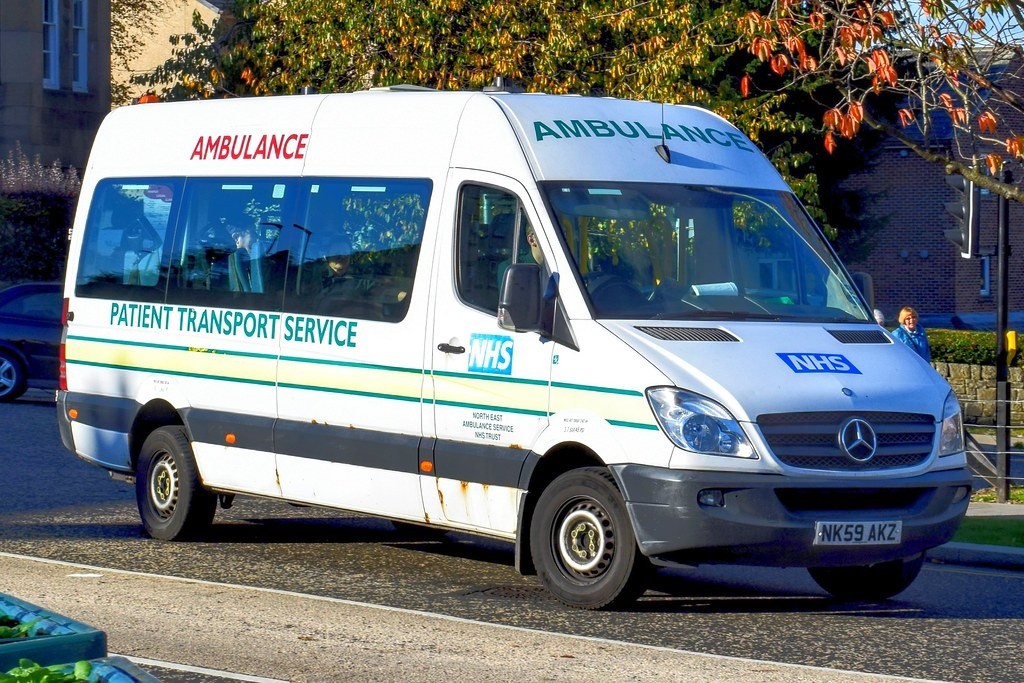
[55,81,973,615]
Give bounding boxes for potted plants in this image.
[0,592,159,683]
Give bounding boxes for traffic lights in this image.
[944,167,980,258]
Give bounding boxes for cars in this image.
[0,279,64,405]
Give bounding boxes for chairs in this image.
[470,213,531,312]
[250,241,270,293]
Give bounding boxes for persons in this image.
[213,221,259,291]
[891,307,931,363]
[312,237,370,301]
[498,221,550,303]
